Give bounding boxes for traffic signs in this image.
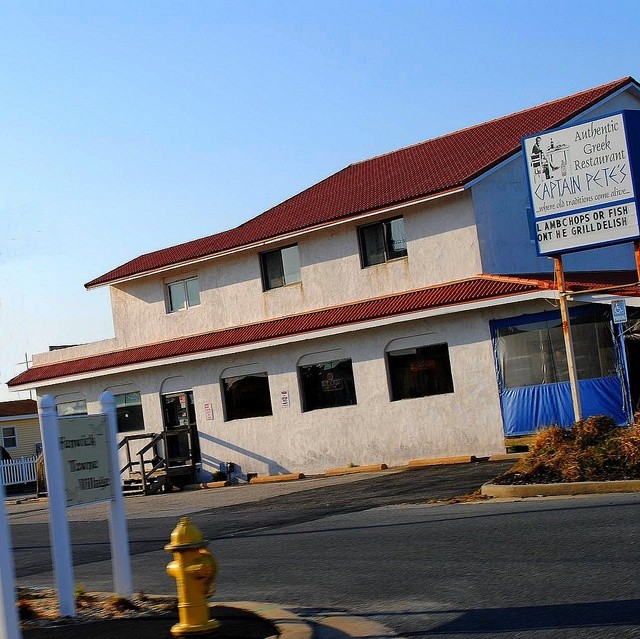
[611,299,627,324]
[521,109,639,256]
